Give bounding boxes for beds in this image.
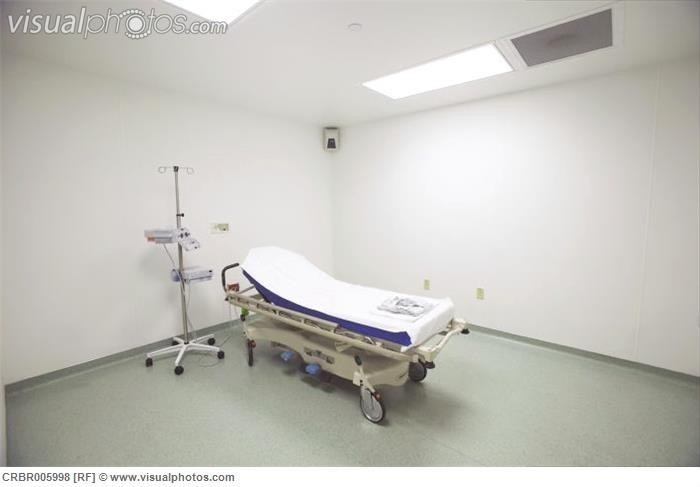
[220,246,468,424]
